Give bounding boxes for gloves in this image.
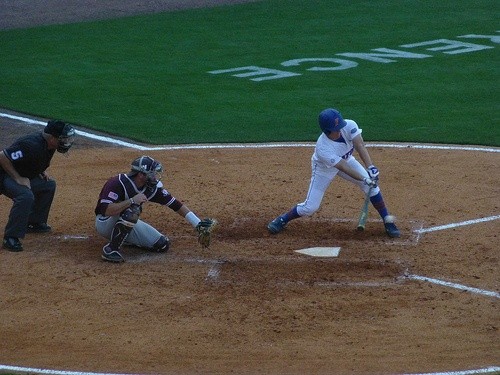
[363,177,377,188]
[367,165,380,181]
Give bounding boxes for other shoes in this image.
[28,223,50,232]
[3,237,23,251]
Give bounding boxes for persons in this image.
[94,156,217,262]
[267,109,401,237]
[0,120,75,252]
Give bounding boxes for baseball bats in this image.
[355,176,379,233]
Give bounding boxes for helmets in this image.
[131,155,163,192]
[319,109,346,131]
[45,121,76,154]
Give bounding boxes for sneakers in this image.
[103,251,124,262]
[384,215,401,238]
[268,215,287,232]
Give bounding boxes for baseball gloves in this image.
[197,218,214,250]
